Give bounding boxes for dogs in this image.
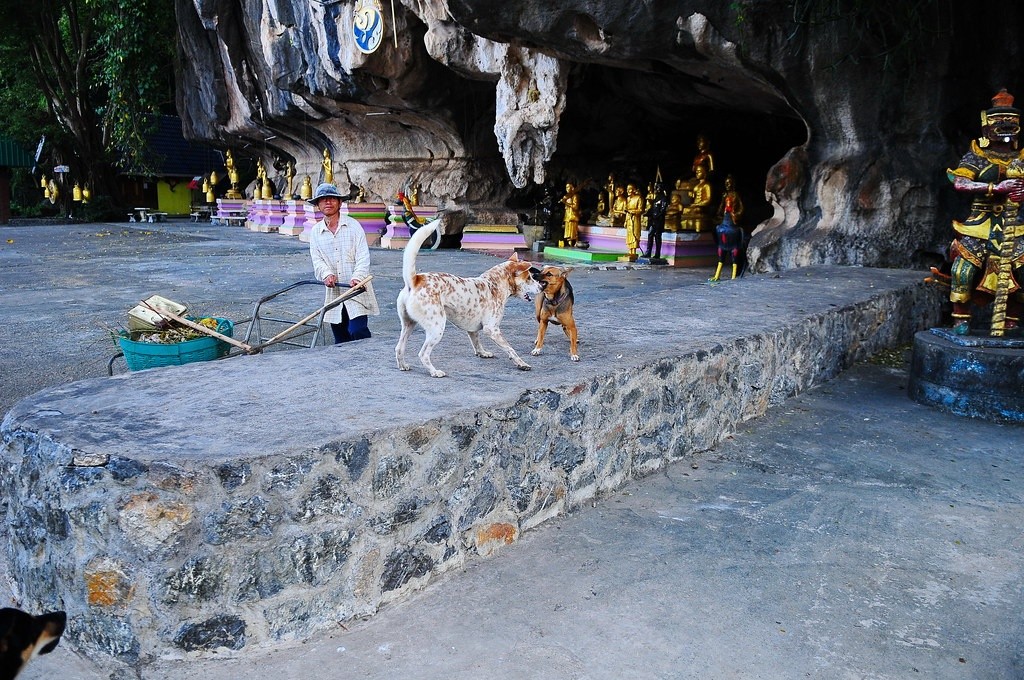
[532,265,580,362]
[396,220,542,379]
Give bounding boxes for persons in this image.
[709,174,743,282]
[641,184,656,230]
[691,132,715,179]
[323,147,336,185]
[283,161,293,194]
[224,151,238,189]
[664,194,683,233]
[624,184,643,254]
[680,164,712,234]
[252,159,270,189]
[948,90,1024,340]
[306,183,381,344]
[559,183,579,248]
[596,175,626,228]
[641,195,666,259]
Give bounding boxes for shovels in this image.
[128,294,259,355]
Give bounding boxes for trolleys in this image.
[106,279,364,379]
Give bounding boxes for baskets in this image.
[119,316,233,371]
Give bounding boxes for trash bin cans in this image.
[118,315,232,373]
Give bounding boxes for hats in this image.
[307,183,347,206]
